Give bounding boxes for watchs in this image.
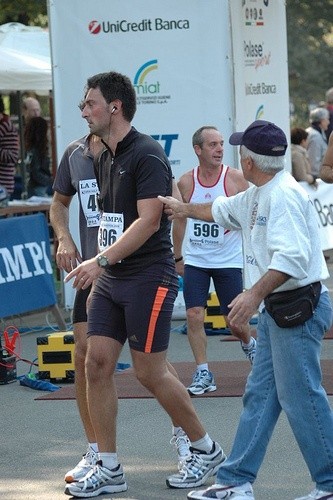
[95,253,111,272]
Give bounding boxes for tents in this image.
[0,22,53,201]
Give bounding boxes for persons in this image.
[172,126,257,395]
[0,90,53,218]
[290,87,333,262]
[49,71,227,497]
[157,120,333,500]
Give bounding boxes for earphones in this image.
[111,106,117,113]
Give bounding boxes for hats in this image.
[229,120,287,156]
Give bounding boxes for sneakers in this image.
[64,446,100,483]
[171,428,191,462]
[185,370,216,395]
[187,481,255,500]
[165,440,228,488]
[295,488,333,500]
[63,459,127,497]
[240,336,257,364]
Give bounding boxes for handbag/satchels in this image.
[264,281,321,329]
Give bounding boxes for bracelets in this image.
[173,256,183,263]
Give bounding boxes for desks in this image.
[0,201,58,282]
